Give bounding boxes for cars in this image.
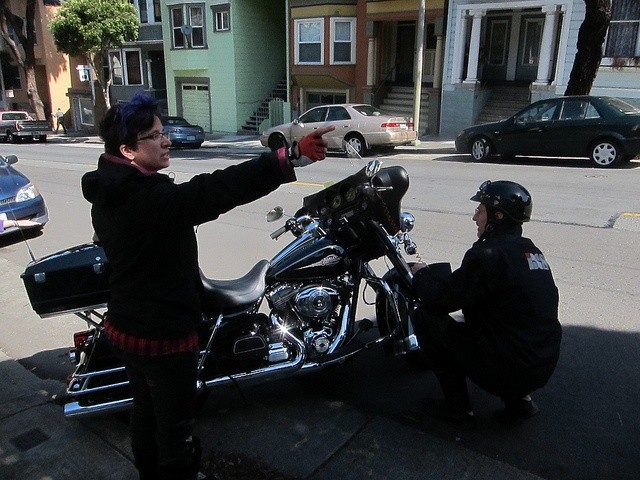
[455,95,640,168]
[0,154,48,236]
[259,104,417,158]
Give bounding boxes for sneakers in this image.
[502,396,540,418]
[423,395,478,431]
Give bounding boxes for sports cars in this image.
[159,116,205,149]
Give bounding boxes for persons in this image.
[408,179,563,419]
[79,91,335,478]
[54,107,68,134]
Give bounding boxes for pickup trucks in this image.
[0,109,52,144]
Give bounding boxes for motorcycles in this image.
[20,137,427,419]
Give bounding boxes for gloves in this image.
[288,124,335,168]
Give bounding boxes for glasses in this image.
[479,180,491,196]
[135,130,170,142]
[475,208,486,214]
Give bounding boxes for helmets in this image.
[469,181,532,222]
